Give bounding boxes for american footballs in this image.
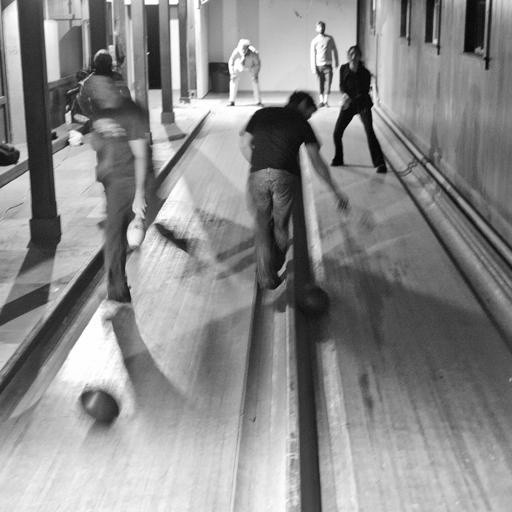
[298,285,329,314]
[81,391,119,421]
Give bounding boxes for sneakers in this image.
[377,163,386,173]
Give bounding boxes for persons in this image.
[309,20,339,108]
[68,74,150,308]
[75,49,131,229]
[225,38,263,106]
[64,69,91,124]
[331,44,387,174]
[238,89,351,293]
